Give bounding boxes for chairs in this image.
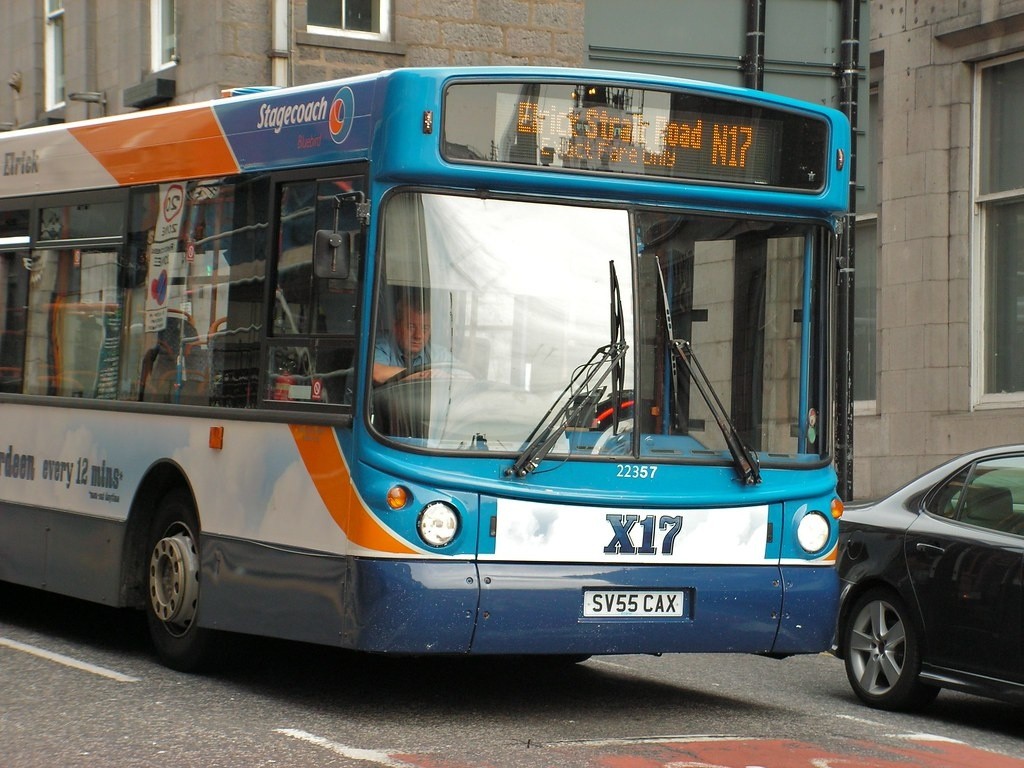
[963,487,1013,531]
[0,301,228,405]
[315,326,355,405]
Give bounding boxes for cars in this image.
[822,443,1024,714]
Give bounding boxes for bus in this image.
[0,63,855,674]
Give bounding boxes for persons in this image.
[344,291,473,406]
